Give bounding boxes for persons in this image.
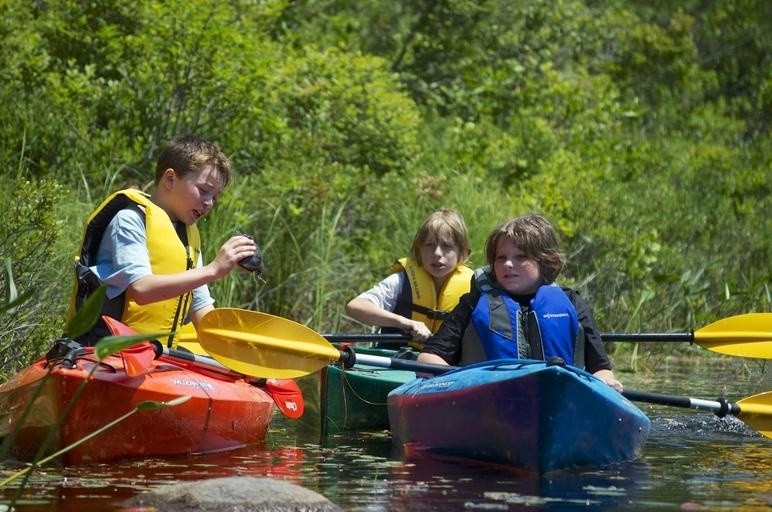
[71,136,257,357]
[345,207,477,351]
[414,214,624,395]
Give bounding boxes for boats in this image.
[385,354,652,479]
[292,360,420,439]
[319,342,417,435]
[389,360,651,464]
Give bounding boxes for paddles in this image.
[99,313,305,420]
[179,312,771,360]
[197,307,772,441]
